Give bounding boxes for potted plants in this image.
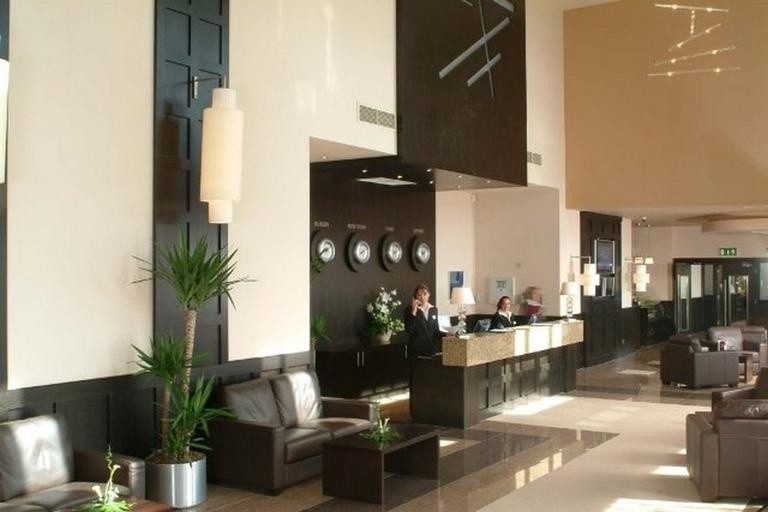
[129,223,250,511]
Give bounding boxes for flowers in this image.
[364,286,403,334]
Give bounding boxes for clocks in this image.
[310,227,433,274]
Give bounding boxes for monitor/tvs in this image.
[473,319,491,331]
[593,237,616,278]
[528,314,541,324]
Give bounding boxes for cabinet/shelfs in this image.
[382,331,416,390]
[314,340,389,401]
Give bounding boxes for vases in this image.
[375,328,393,344]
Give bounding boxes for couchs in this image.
[1,407,146,512]
[686,364,767,503]
[193,371,383,496]
[659,323,767,391]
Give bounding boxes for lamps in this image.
[569,253,601,298]
[624,257,651,293]
[188,75,246,224]
[559,281,582,321]
[449,285,476,338]
[622,215,654,265]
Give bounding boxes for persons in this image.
[489,296,518,328]
[403,283,442,420]
[521,286,543,321]
